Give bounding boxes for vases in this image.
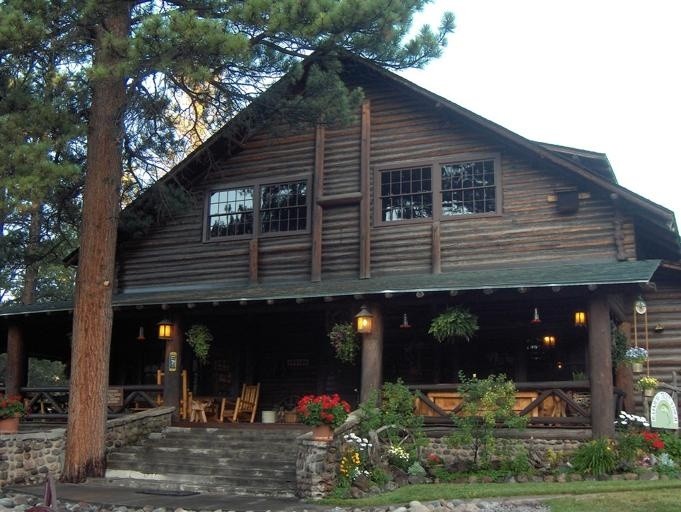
[312,423,332,440]
[632,362,643,373]
[644,388,654,397]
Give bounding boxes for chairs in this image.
[157,370,207,423]
[220,382,261,424]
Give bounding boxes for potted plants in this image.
[428,303,478,343]
[0,413,21,433]
[186,325,214,366]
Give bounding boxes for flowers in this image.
[328,322,360,365]
[296,393,351,428]
[638,376,658,389]
[624,347,648,363]
[0,392,29,419]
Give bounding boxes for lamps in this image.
[157,319,173,340]
[542,334,557,349]
[355,305,374,334]
[573,310,586,328]
[557,183,579,215]
